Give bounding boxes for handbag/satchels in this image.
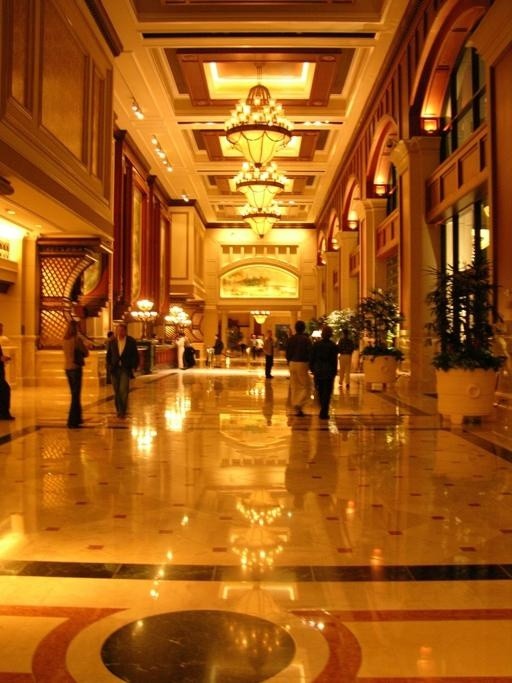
[73,346,86,366]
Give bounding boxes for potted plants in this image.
[423,257,507,424]
[350,287,405,390]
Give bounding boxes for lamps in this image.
[164,305,192,339]
[224,61,293,168]
[250,310,270,324]
[241,202,281,239]
[235,161,285,211]
[131,299,158,339]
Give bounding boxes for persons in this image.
[239,321,354,420]
[105,332,115,384]
[63,317,89,428]
[0,322,15,420]
[174,332,194,369]
[213,335,223,368]
[152,335,160,344]
[107,324,139,419]
